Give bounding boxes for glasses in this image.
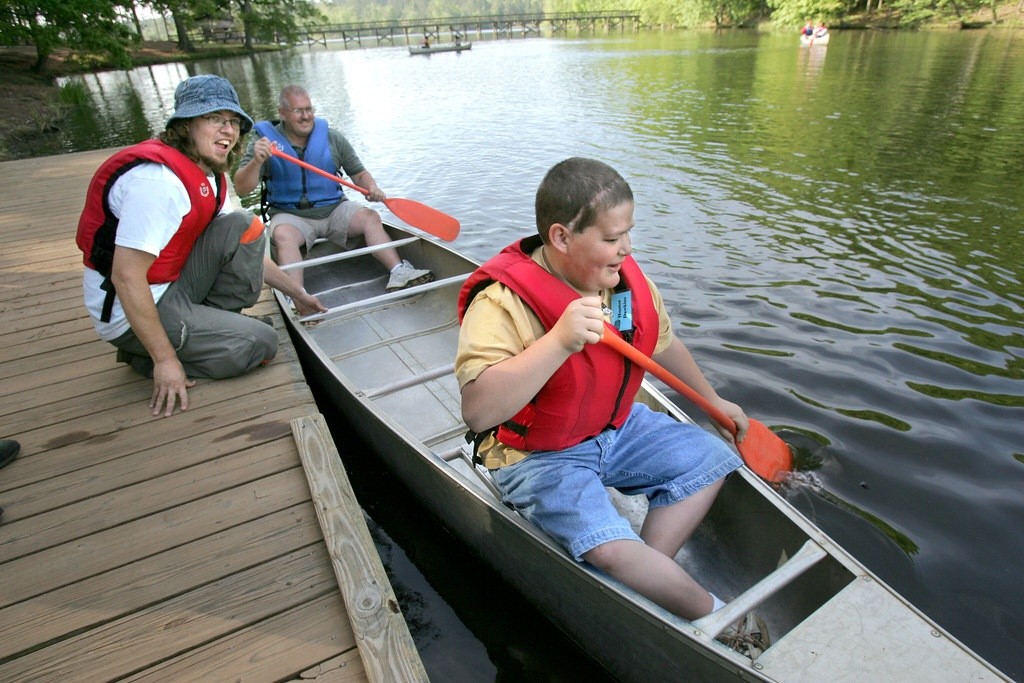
[283,105,315,117]
[201,112,245,130]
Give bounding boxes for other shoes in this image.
[113,348,135,364]
[0,439,21,468]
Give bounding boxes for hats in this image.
[167,75,254,136]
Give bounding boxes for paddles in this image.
[599,321,795,484]
[269,142,461,242]
[809,22,819,48]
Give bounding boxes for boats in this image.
[800,32,830,47]
[261,206,1021,683]
[961,20,992,30]
[408,41,473,54]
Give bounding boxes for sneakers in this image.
[385,258,436,293]
[286,287,307,314]
[717,611,770,661]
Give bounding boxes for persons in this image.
[456,158,750,619]
[455,32,461,46]
[76,75,330,417]
[806,20,825,37]
[422,36,429,48]
[234,84,435,309]
